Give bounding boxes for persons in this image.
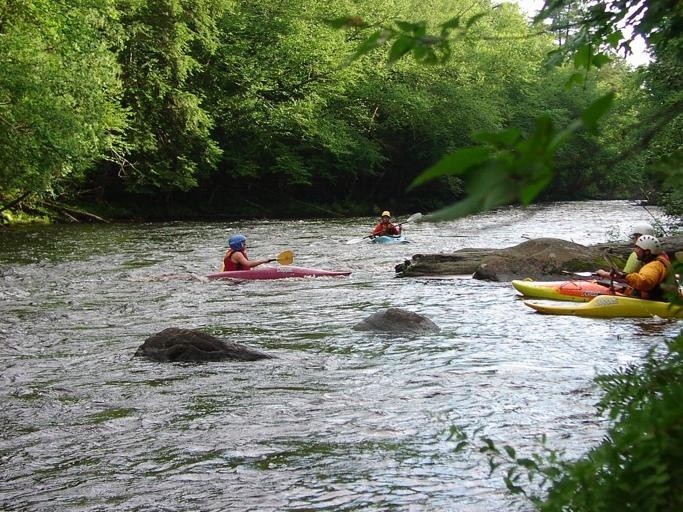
[224,235,276,270]
[595,223,681,300]
[373,210,402,238]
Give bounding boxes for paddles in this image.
[345,213,422,244]
[561,270,597,277]
[220,250,293,272]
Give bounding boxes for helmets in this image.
[228,235,246,250]
[381,211,390,217]
[633,224,660,255]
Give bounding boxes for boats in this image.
[510,251,683,318]
[199,265,355,279]
[371,236,395,244]
